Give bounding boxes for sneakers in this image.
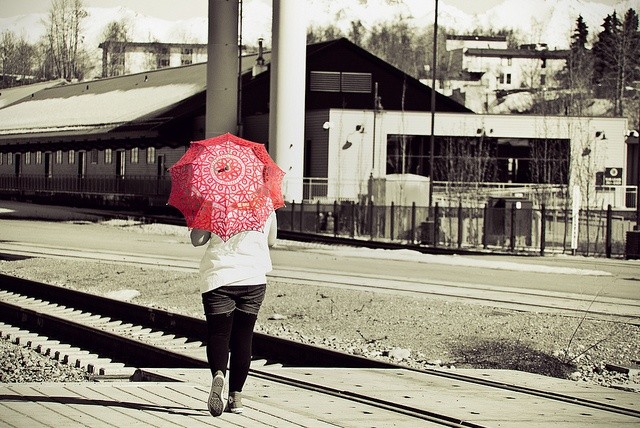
[223,391,244,413]
[208,370,225,417]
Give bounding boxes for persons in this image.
[189,191,278,417]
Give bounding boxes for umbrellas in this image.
[165,132,287,244]
